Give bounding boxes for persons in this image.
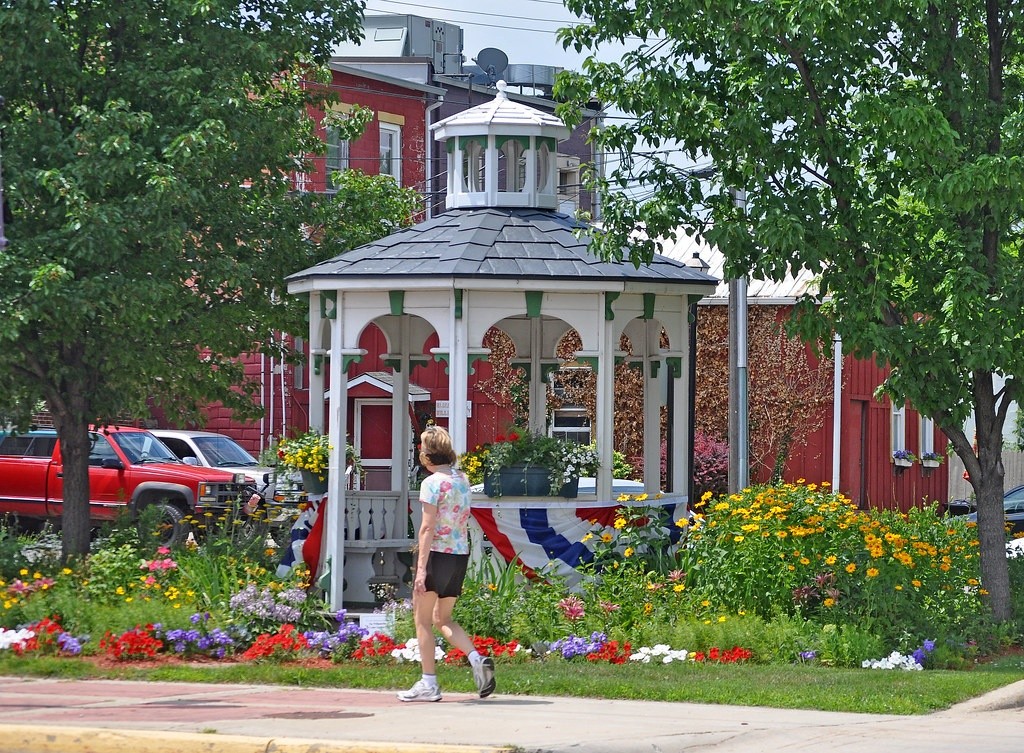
[393,424,500,706]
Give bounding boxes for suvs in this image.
[143,428,313,516]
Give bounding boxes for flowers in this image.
[258,426,365,482]
[1,493,1024,666]
[630,431,730,489]
[457,425,604,483]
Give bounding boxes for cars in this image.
[938,484,1024,538]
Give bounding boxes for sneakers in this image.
[473,655,496,698]
[395,681,442,703]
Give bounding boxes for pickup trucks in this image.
[0,419,258,549]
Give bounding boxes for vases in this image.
[299,466,329,494]
[482,461,582,498]
